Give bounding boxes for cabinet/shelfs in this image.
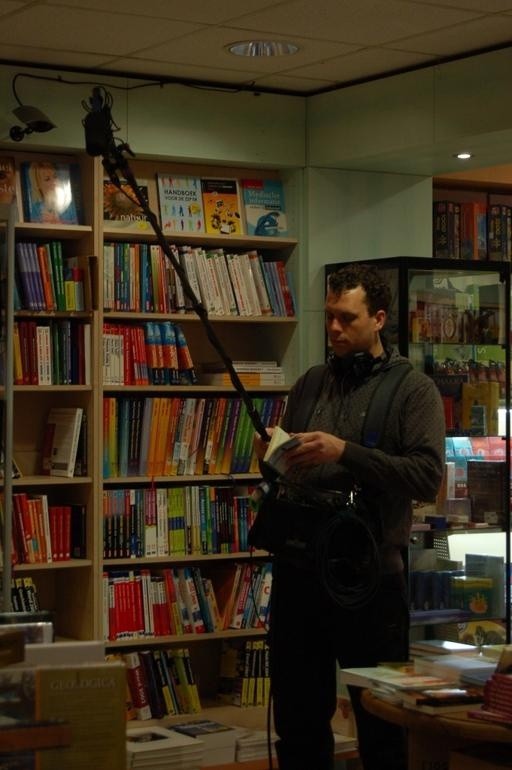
[0,142,512,718]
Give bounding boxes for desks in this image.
[362,689,512,770]
[0,721,72,755]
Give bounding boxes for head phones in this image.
[337,351,383,383]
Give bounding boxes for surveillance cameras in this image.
[11,103,56,143]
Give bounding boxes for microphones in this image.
[82,87,114,154]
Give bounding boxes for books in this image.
[24,641,106,667]
[334,733,358,754]
[11,491,87,611]
[105,641,279,770]
[37,409,88,478]
[2,321,285,386]
[433,200,512,261]
[0,241,296,318]
[338,282,512,725]
[103,398,285,479]
[1,622,52,727]
[264,425,321,486]
[2,438,23,479]
[103,486,272,641]
[1,159,288,236]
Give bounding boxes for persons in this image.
[253,266,444,770]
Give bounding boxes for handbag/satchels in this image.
[248,496,345,568]
